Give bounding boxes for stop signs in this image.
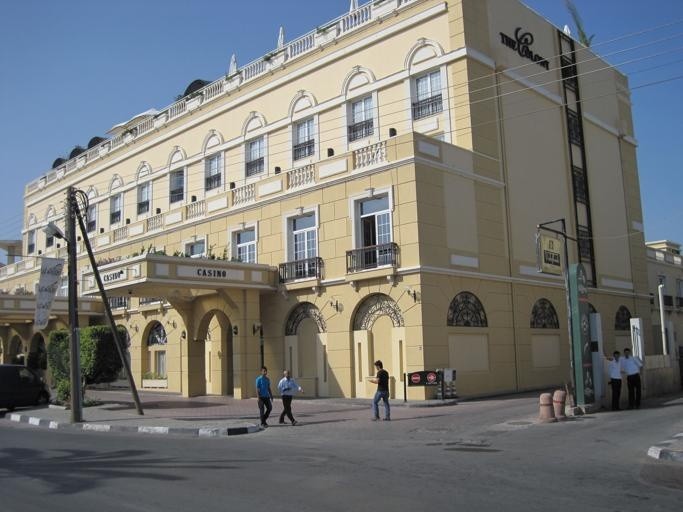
[410,374,420,384]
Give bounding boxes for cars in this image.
[0,363,48,413]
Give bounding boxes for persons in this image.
[276,369,304,427]
[607,350,624,410]
[366,359,390,421]
[619,346,645,409]
[253,367,276,428]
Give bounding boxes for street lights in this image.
[41,219,83,424]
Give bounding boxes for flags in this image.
[31,256,64,332]
